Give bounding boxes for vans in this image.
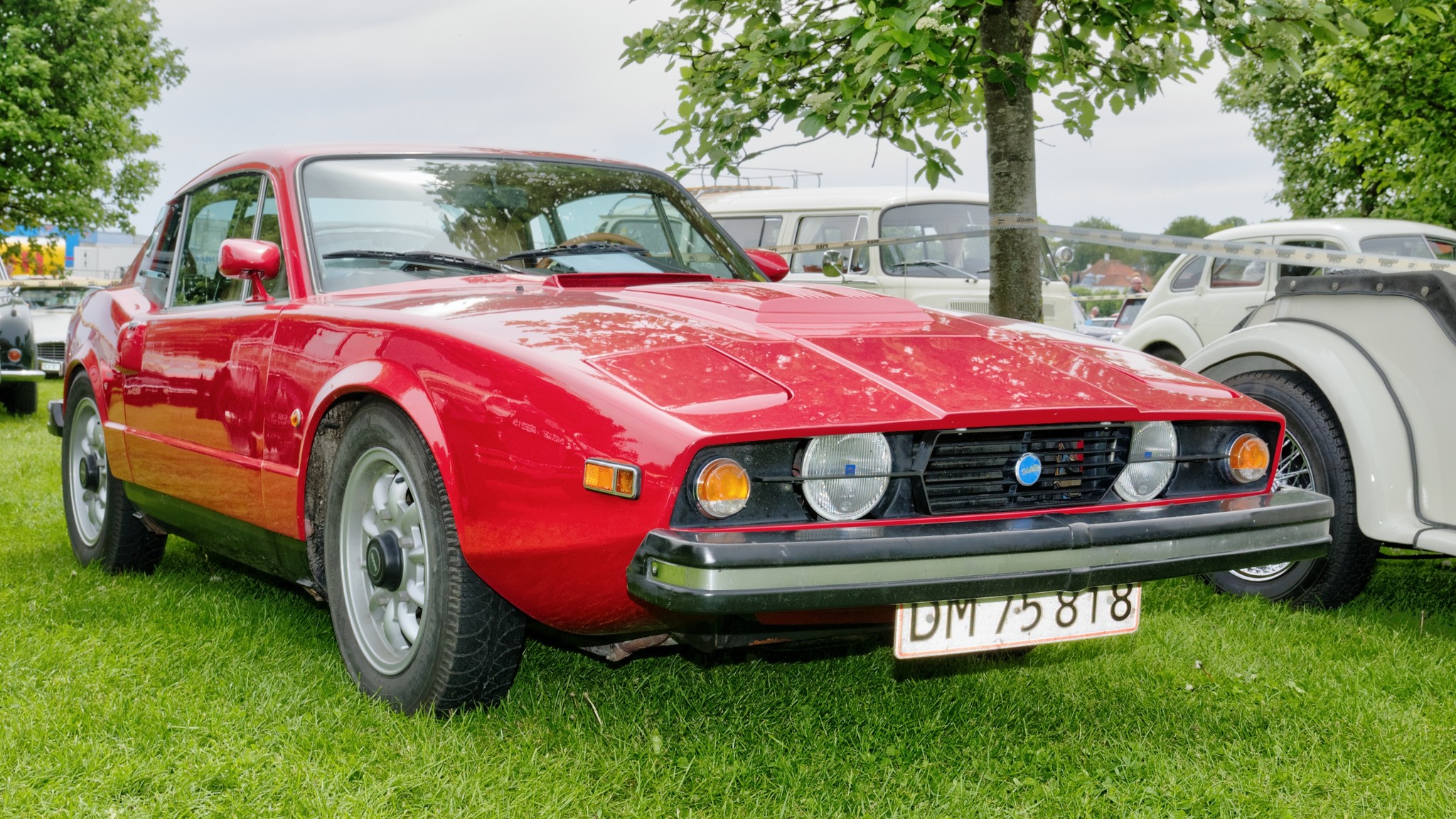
[590,162,1088,333]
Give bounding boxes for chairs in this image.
[449,219,526,270]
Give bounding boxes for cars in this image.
[1076,214,1456,616]
[0,239,122,418]
[40,146,1336,721]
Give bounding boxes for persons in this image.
[1131,273,1141,278]
[1123,277,1147,303]
[1241,260,1265,282]
[1059,275,1080,305]
[1090,306,1100,318]
